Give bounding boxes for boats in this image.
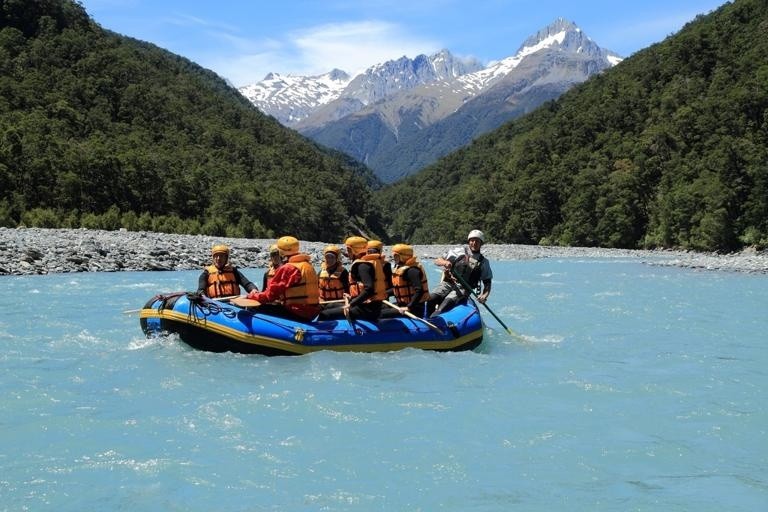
[136,290,488,359]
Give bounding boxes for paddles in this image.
[231,298,261,307]
[382,299,444,335]
[344,297,351,324]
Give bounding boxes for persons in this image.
[246,235,430,325]
[427,230,493,319]
[196,244,258,303]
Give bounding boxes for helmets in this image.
[391,243,414,263]
[274,235,300,256]
[268,243,280,257]
[211,245,229,258]
[345,236,368,255]
[367,239,384,257]
[467,229,485,243]
[323,245,341,257]
